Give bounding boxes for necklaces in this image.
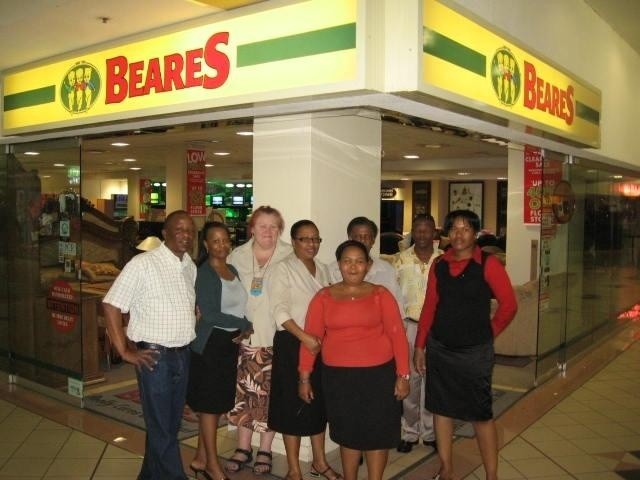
[350,297,355,301]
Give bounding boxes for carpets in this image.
[453,388,527,439]
[82,374,234,440]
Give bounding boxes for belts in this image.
[133,342,190,351]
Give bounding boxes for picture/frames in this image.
[449,180,484,229]
[411,180,431,220]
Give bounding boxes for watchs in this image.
[395,373,410,381]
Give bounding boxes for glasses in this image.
[294,237,320,244]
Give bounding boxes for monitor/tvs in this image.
[211,195,226,207]
[232,196,246,206]
[250,195,253,208]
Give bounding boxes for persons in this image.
[413,210,518,480]
[265,220,343,480]
[225,207,294,475]
[298,240,412,479]
[100,210,198,480]
[329,216,405,327]
[391,214,447,454]
[189,223,255,479]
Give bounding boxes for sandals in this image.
[309,465,343,479]
[223,448,250,475]
[253,449,271,475]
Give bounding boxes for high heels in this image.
[186,463,203,476]
[203,468,228,480]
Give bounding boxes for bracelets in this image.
[298,378,312,384]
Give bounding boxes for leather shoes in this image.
[422,442,437,448]
[397,438,416,453]
